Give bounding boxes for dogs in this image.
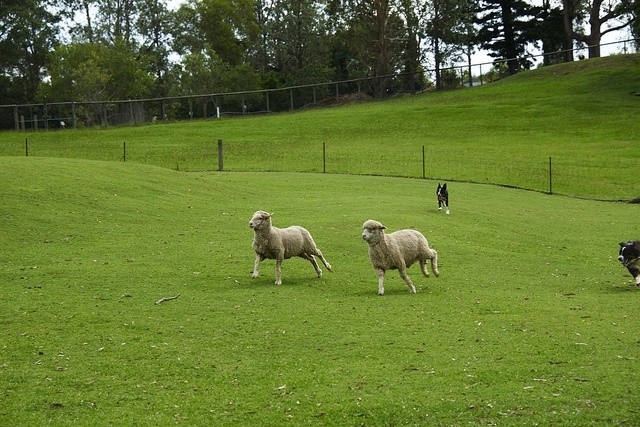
[436,183,449,215]
[618,240,640,286]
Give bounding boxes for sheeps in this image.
[249,210,333,286]
[362,219,439,297]
